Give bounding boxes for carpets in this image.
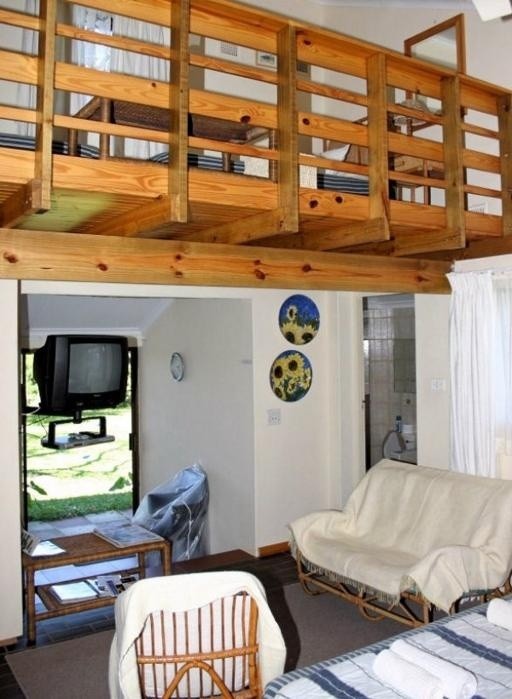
[5,581,483,699]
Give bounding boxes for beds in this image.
[263,591,512,699]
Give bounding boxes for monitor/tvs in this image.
[34,334,129,416]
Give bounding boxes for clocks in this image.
[168,353,185,382]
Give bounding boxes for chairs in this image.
[343,114,394,197]
[108,571,286,698]
[394,153,444,207]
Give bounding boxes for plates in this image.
[270,350,312,402]
[279,294,320,345]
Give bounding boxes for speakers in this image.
[472,0,512,23]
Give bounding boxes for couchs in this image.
[286,458,512,627]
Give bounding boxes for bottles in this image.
[402,425,416,450]
[395,416,402,432]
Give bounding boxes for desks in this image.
[66,72,247,171]
[22,531,171,648]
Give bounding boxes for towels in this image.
[373,648,447,699]
[387,638,479,699]
[487,597,512,630]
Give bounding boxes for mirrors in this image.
[403,13,466,133]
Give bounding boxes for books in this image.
[22,523,165,605]
